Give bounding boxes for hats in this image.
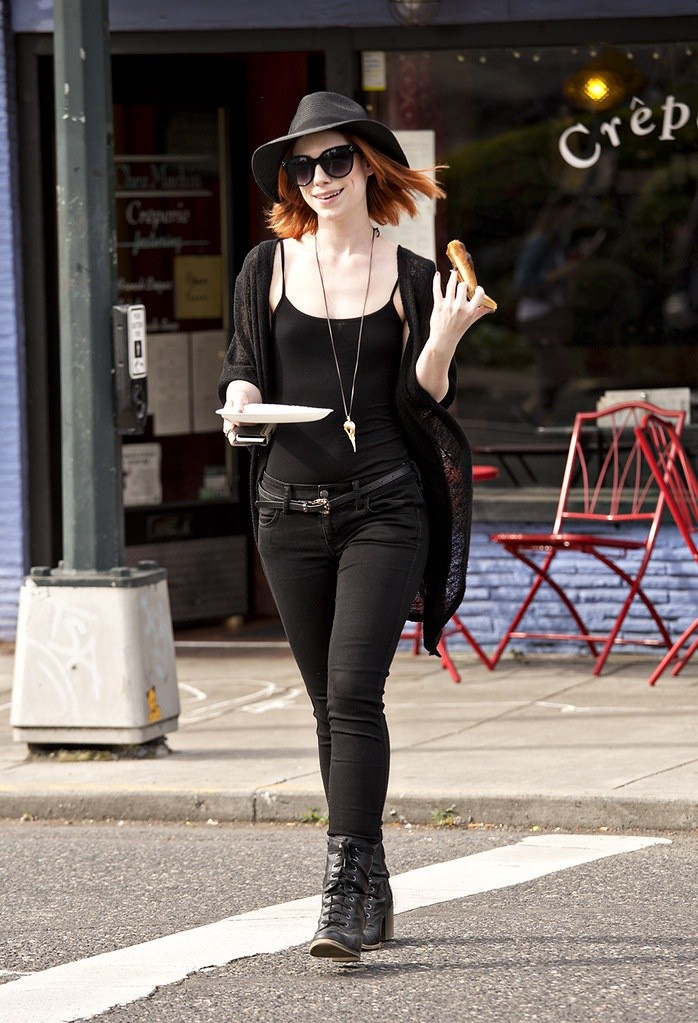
[252,92,410,204]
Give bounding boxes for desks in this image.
[472,444,634,487]
[394,464,500,681]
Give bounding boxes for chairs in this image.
[490,403,685,677]
[630,415,698,687]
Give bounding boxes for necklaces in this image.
[314,226,380,452]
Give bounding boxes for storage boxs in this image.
[152,333,192,436]
[192,330,229,434]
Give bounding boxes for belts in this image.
[255,463,411,514]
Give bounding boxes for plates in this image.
[214,402,334,424]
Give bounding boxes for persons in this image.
[217,93,495,962]
[501,191,698,428]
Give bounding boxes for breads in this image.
[446,239,498,310]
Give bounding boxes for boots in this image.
[362,840,394,949]
[310,835,375,961]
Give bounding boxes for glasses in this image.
[282,144,362,187]
